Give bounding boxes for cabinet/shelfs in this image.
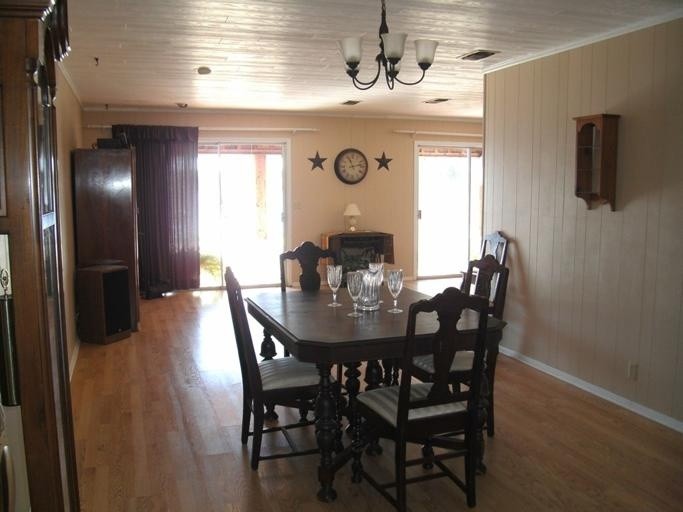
[72,147,140,324]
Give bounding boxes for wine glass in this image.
[385,268,404,313]
[325,264,344,307]
[368,261,384,304]
[345,271,364,318]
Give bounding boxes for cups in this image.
[357,270,380,310]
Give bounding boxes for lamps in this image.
[343,203,361,231]
[335,0,440,91]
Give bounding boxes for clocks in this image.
[333,148,368,185]
